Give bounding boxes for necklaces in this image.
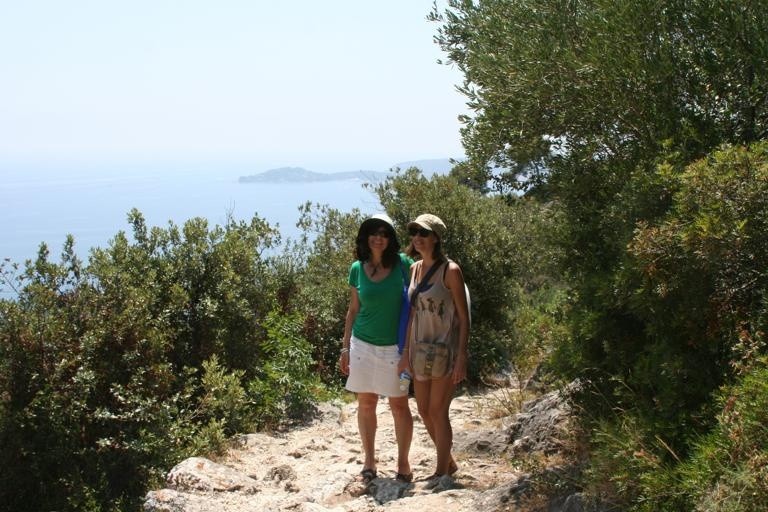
[367,259,386,280]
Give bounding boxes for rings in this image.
[340,368,344,372]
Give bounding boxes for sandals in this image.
[348,469,378,497]
[396,473,413,498]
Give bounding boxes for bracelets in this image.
[402,345,409,353]
[338,347,349,354]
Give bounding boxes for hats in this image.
[358,214,396,240]
[407,213,448,239]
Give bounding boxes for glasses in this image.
[369,230,389,238]
[408,228,430,237]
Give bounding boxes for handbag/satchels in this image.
[412,343,455,382]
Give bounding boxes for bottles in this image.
[399,369,411,391]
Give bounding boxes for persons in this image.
[395,212,473,495]
[336,213,415,498]
